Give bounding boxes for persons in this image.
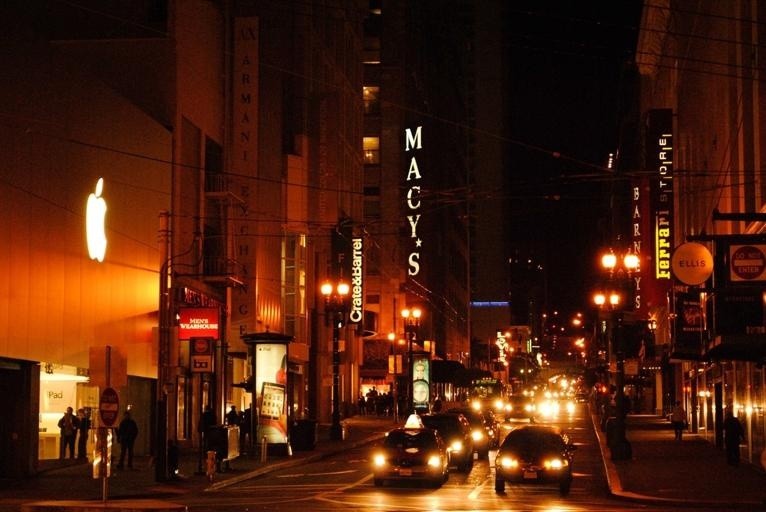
[723,411,745,463]
[55,407,79,464]
[671,400,686,441]
[77,409,89,459]
[116,411,138,470]
[228,405,239,426]
[358,386,393,417]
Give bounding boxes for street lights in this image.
[400,308,422,418]
[601,251,640,467]
[590,290,622,400]
[316,280,356,444]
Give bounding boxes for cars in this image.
[494,423,576,497]
[371,413,453,490]
[415,411,477,476]
[442,376,579,460]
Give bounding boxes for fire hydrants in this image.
[202,449,217,484]
